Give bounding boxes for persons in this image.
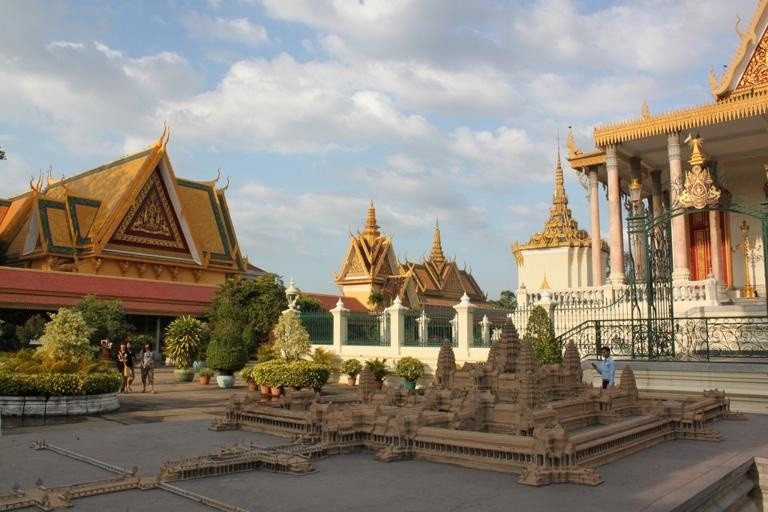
[592,347,615,389]
[115,341,137,394]
[139,341,156,394]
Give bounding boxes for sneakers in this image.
[141,389,156,393]
[119,387,134,394]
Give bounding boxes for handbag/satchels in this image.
[124,366,130,376]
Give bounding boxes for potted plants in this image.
[239,356,331,396]
[205,296,248,388]
[339,355,425,389]
[161,313,202,383]
[199,367,214,385]
